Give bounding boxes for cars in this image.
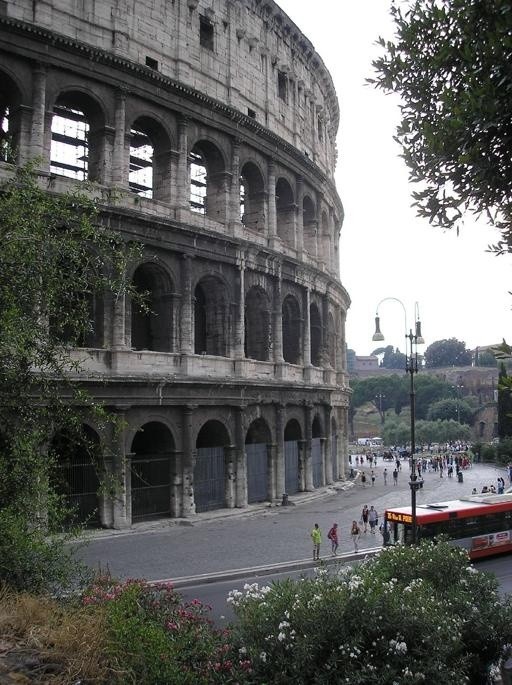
[365,447,419,460]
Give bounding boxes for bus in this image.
[380,493,511,561]
[357,437,371,446]
[370,437,384,449]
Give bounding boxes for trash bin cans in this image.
[458,471,464,482]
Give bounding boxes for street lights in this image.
[372,296,424,543]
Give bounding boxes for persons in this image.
[393,468,398,486]
[326,523,340,557]
[383,468,388,485]
[348,439,475,482]
[496,477,504,494]
[504,461,512,489]
[370,470,375,487]
[360,504,369,533]
[368,505,377,535]
[350,520,361,554]
[378,519,393,548]
[472,487,479,495]
[310,523,322,561]
[481,486,489,493]
[360,471,366,487]
[500,477,505,489]
